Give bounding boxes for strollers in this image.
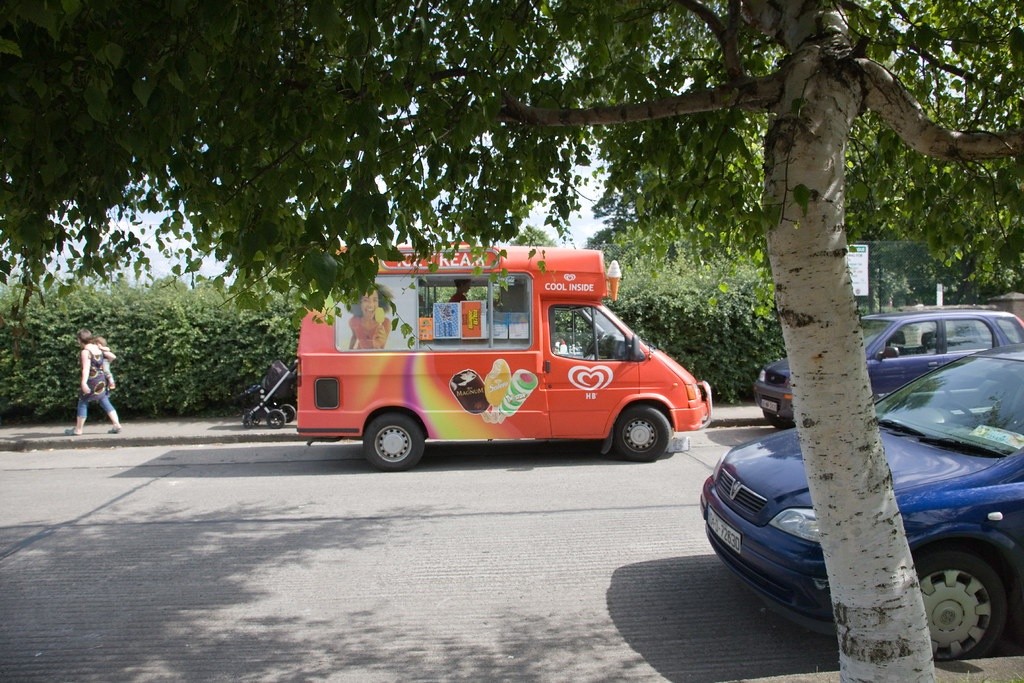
[235,357,297,429]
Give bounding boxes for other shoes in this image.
[109,383,116,389]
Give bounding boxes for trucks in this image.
[296,244,712,470]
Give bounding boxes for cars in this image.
[700,342,1024,661]
[753,307,1024,429]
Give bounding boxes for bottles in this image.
[554,341,575,354]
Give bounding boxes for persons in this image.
[65,328,123,436]
[346,283,397,350]
[448,279,471,303]
[94,336,117,391]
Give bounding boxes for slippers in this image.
[65,428,81,435]
[108,429,121,433]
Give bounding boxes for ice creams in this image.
[607,259,621,300]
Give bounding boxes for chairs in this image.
[915,332,937,353]
[886,331,908,355]
